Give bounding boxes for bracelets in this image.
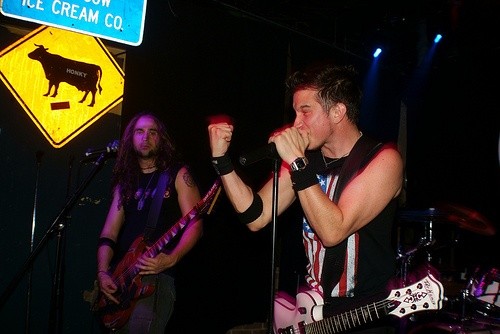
[97,270,111,275]
[212,155,234,176]
[292,167,319,191]
[95,238,116,252]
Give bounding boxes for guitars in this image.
[79,155,246,334]
[273,261,447,334]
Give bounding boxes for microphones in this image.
[80,145,119,161]
[240,142,279,166]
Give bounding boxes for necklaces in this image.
[139,165,158,171]
[136,172,156,211]
[318,131,365,168]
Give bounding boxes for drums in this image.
[463,267,500,317]
[441,276,467,296]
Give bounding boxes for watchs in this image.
[290,157,310,171]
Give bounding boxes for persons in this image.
[207,63,406,334]
[95,112,204,334]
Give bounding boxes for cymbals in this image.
[398,207,446,223]
[439,203,496,235]
